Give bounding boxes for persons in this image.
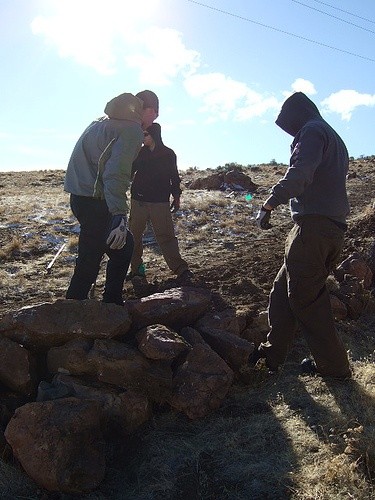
[125,123,194,279]
[63,90,160,306]
[250,91,353,378]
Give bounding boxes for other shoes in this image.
[250,346,276,372]
[177,270,189,282]
[300,358,320,374]
[124,272,144,280]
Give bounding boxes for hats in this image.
[137,91,158,114]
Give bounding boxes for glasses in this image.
[143,133,151,137]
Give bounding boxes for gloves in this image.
[170,193,180,213]
[106,215,127,249]
[256,205,273,230]
